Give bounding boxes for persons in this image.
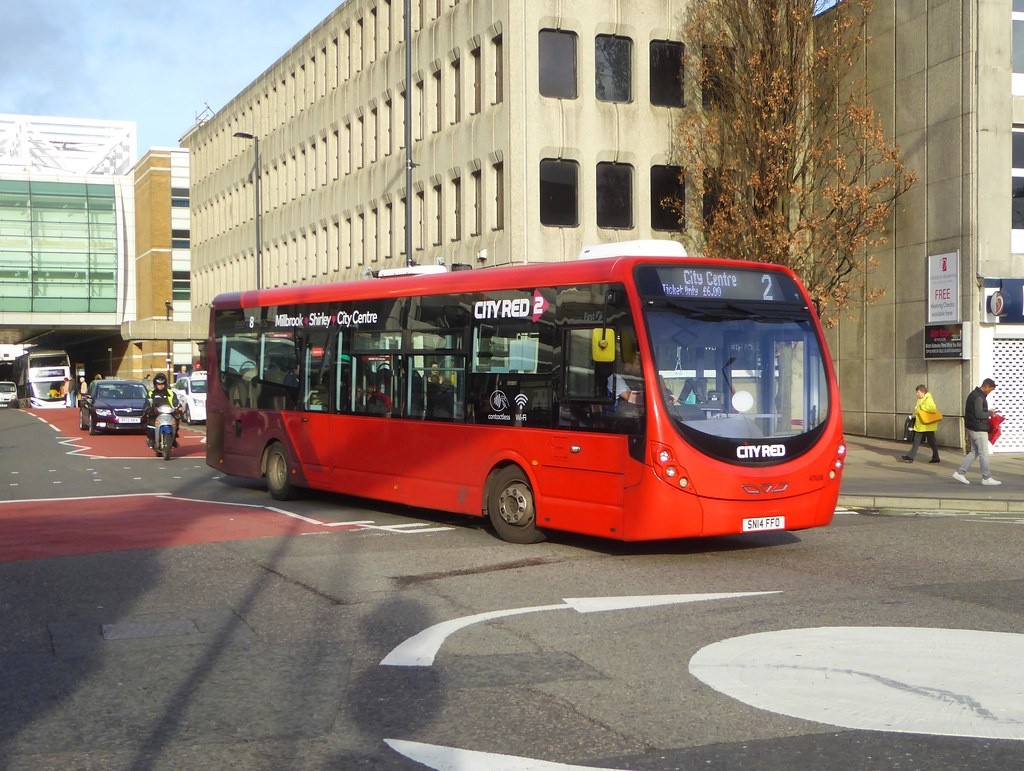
[607,342,678,409]
[144,374,150,379]
[177,365,189,380]
[286,361,455,416]
[952,379,1001,485]
[80,377,87,393]
[49,387,59,397]
[63,375,76,408]
[143,373,184,449]
[900,385,940,462]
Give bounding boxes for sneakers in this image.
[953,470,969,484]
[982,477,1002,486]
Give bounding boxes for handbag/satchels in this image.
[903,414,926,442]
[989,414,1006,445]
[918,398,943,425]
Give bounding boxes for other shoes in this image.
[929,460,940,463]
[148,439,153,448]
[171,439,178,447]
[901,455,913,463]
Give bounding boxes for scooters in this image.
[147,400,184,461]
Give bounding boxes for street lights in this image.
[233,133,260,290]
[165,300,171,387]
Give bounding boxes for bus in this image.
[12,349,71,408]
[206,240,847,546]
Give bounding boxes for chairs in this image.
[238,361,271,408]
[378,362,392,379]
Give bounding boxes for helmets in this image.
[154,376,166,385]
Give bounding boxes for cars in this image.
[172,376,207,426]
[0,382,17,408]
[79,379,149,436]
